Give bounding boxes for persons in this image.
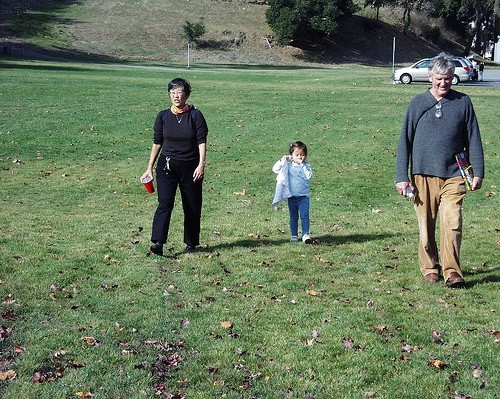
[271,141,313,244]
[139,78,208,255]
[395,52,485,287]
[472,59,484,81]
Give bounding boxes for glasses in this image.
[166,90,184,96]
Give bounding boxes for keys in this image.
[163,157,171,175]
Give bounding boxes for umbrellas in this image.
[466,54,480,60]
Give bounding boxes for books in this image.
[455,151,475,191]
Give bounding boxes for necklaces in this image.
[176,113,184,124]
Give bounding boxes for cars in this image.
[394,58,470,85]
[433,56,475,78]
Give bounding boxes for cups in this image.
[141,175,155,194]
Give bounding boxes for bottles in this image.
[395,181,415,200]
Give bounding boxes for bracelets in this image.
[199,161,206,165]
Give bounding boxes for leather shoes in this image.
[444,272,466,287]
[424,271,438,284]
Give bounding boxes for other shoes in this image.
[148,244,163,255]
[291,234,299,243]
[186,245,194,254]
[304,235,313,246]
